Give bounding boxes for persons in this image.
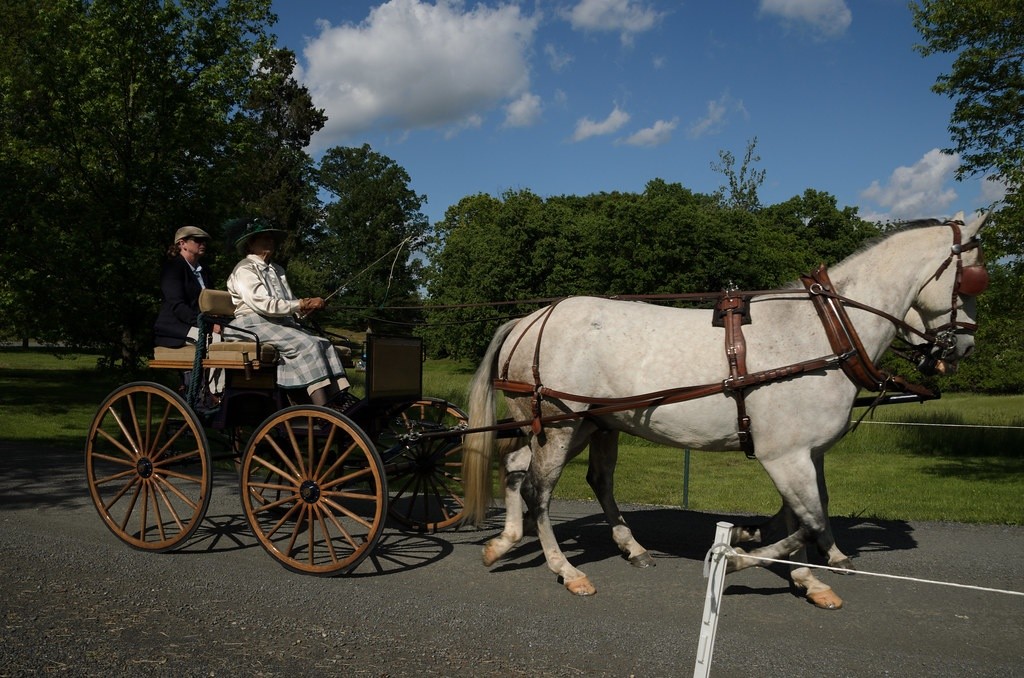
[155,226,225,404]
[227,218,351,430]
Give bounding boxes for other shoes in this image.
[316,397,360,429]
[209,392,223,406]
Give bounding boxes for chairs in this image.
[199,289,351,434]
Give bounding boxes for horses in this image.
[459,204,996,610]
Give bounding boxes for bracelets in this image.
[303,298,311,309]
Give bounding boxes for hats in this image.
[174,225,209,245]
[234,216,289,246]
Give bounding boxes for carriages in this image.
[84,210,1001,609]
